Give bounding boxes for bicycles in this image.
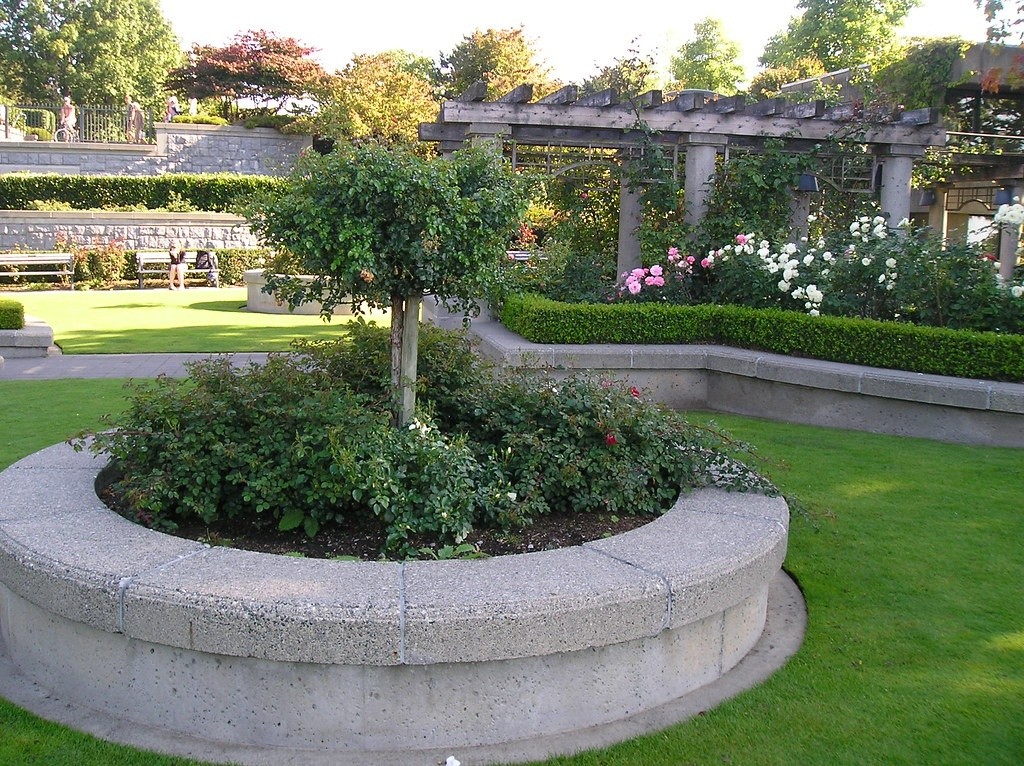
[55,118,79,143]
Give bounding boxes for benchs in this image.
[0,253,74,292]
[134,252,221,290]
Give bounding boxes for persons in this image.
[130,101,147,145]
[124,95,137,145]
[164,95,180,122]
[167,239,189,291]
[59,96,77,139]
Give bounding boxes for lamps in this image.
[918,191,936,206]
[795,173,820,193]
[992,190,1012,205]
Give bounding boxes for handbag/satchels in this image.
[195,250,218,269]
